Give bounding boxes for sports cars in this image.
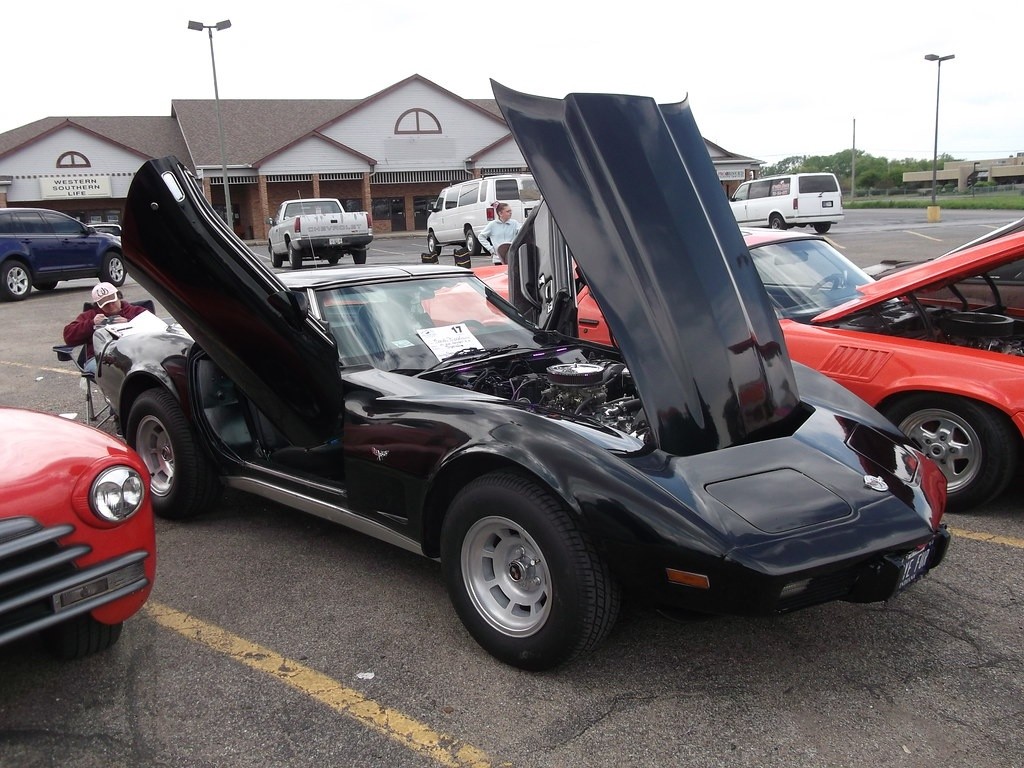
[411,216,1024,514]
[103,78,952,671]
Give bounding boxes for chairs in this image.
[420,252,439,265]
[454,247,472,269]
[51,300,157,430]
[357,299,421,350]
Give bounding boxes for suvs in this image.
[0,207,128,303]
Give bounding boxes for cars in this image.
[84,221,123,241]
[0,402,155,655]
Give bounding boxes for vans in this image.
[728,172,844,235]
[426,173,543,256]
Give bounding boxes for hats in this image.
[92,282,119,308]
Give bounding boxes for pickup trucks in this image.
[265,198,374,270]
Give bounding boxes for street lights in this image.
[187,19,232,228]
[925,53,956,206]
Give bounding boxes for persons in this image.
[478,203,521,265]
[63,283,147,438]
